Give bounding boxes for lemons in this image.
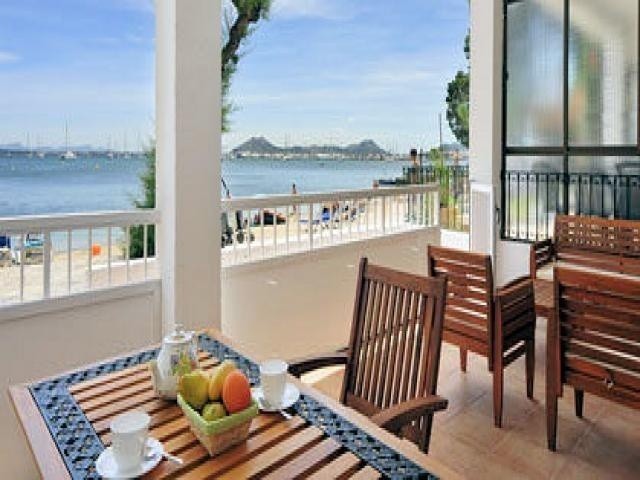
[148,323,200,400]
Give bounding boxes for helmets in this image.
[96,437,164,479]
[250,382,300,412]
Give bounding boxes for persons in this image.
[290,183,297,213]
[409,157,419,184]
[447,148,463,166]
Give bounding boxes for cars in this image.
[259,358,289,406]
[109,412,151,470]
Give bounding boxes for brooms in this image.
[202,401,226,422]
[208,360,235,402]
[223,371,250,413]
[180,372,208,410]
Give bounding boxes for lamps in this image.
[288,256,449,455]
[545,266,640,452]
[427,243,536,429]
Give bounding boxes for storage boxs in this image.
[530,214,640,279]
